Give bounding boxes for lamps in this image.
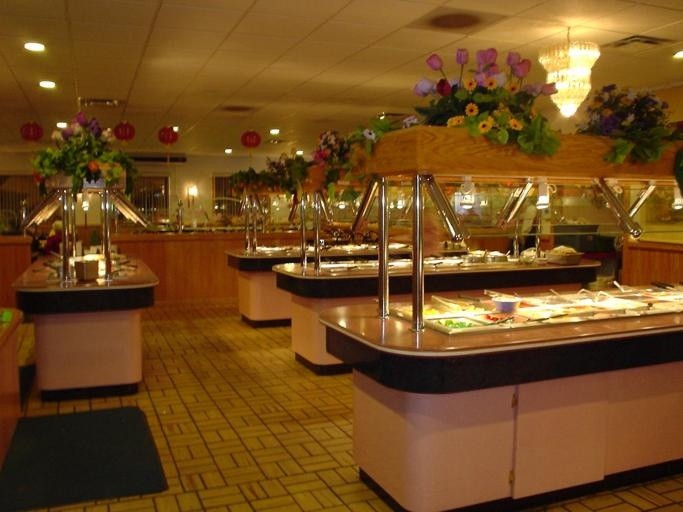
[537,38,602,117]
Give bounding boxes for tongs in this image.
[430,294,470,311]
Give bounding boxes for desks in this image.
[272,257,601,375]
[223,241,468,327]
[0,231,315,303]
[317,282,683,512]
[13,250,159,403]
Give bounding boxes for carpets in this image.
[0,405,169,512]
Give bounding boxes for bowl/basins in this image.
[492,296,521,313]
[545,252,586,264]
